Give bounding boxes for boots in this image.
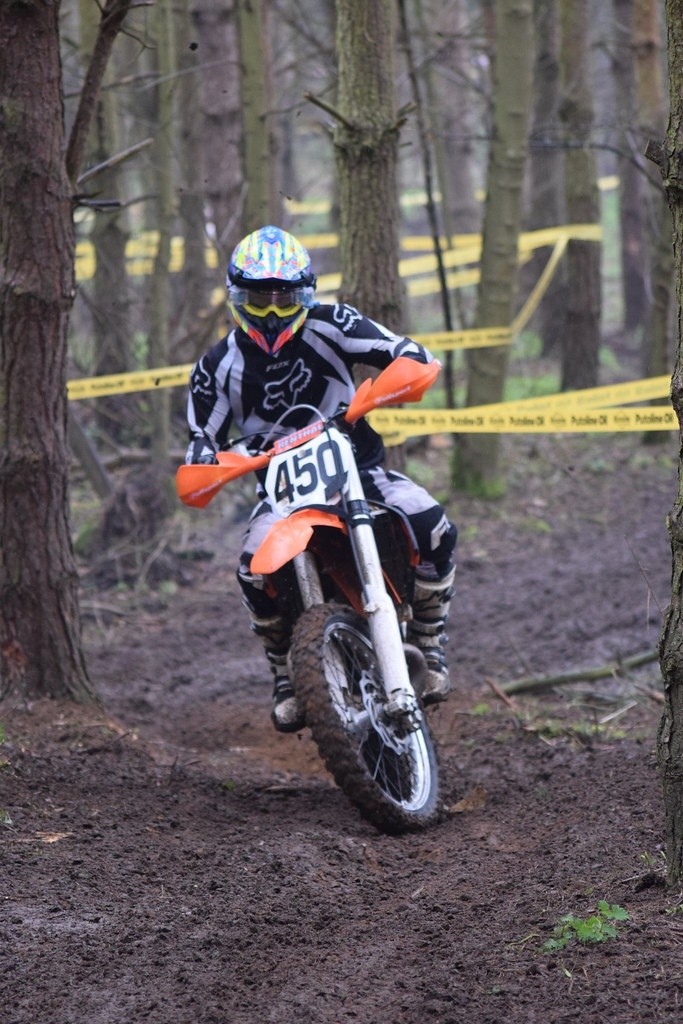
[250,614,307,733]
[404,564,456,704]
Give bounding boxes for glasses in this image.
[241,285,304,318]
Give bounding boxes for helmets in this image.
[226,226,317,356]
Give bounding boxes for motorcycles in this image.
[174,361,454,836]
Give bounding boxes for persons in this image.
[184,225,458,735]
